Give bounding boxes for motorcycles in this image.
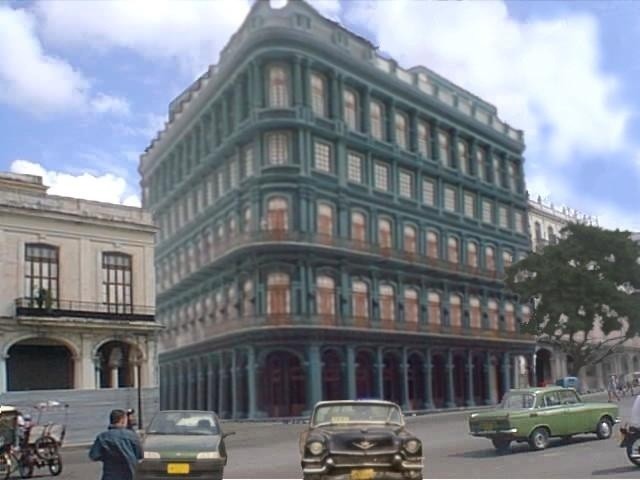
[620,423,640,469]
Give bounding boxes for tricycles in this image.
[0,405,69,480]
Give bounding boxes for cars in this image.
[468,385,620,452]
[298,399,425,480]
[135,410,235,479]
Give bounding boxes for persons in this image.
[89,408,144,480]
[608,376,633,402]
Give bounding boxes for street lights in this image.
[134,333,148,429]
